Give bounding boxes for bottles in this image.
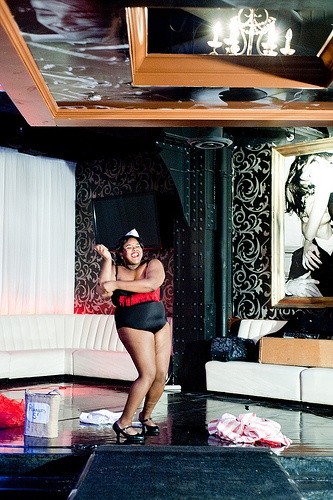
[79,412,114,424]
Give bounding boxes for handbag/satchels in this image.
[23,389,60,438]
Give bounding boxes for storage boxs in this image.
[258,336,333,367]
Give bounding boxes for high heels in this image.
[112,421,144,443]
[138,412,160,432]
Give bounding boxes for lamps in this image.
[284,127,296,142]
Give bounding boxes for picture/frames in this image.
[270,136,333,308]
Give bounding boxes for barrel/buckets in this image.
[23,388,60,438]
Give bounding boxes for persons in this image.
[285,151,333,297]
[94,235,170,441]
[31,0,126,45]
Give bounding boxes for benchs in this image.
[204,319,333,410]
[0,312,171,386]
